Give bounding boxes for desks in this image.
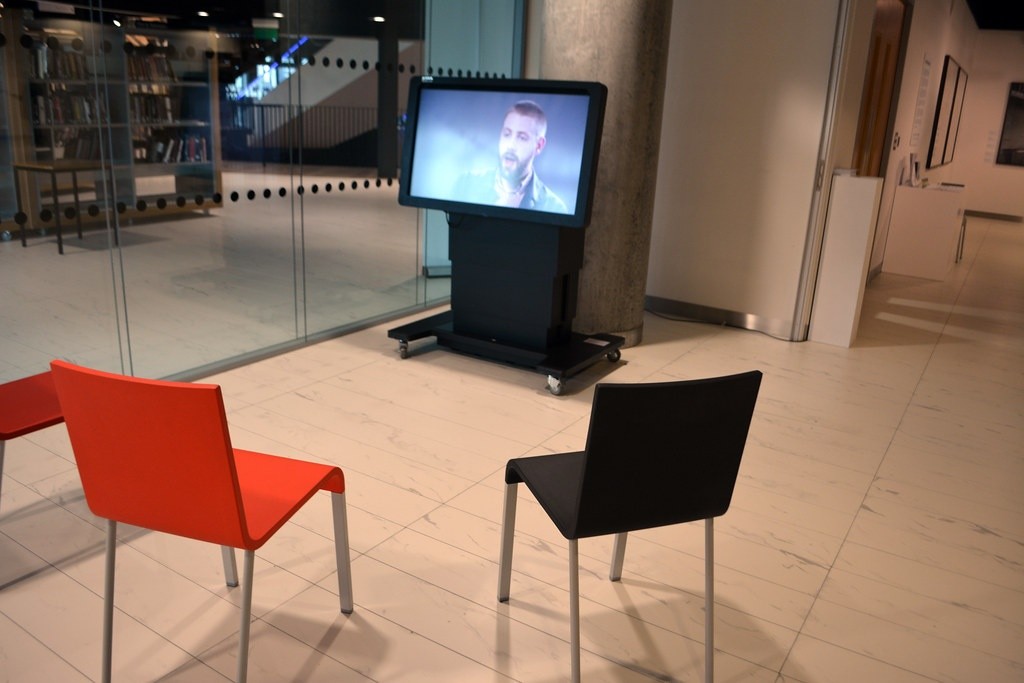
[12,159,121,255]
[880,180,971,282]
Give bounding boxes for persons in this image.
[452,100,566,213]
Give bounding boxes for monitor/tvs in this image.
[397,75,609,228]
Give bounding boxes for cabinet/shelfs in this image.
[7,45,224,233]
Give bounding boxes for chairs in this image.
[497,368,763,683]
[50,359,353,683]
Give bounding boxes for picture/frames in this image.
[995,81,1024,166]
[926,54,969,170]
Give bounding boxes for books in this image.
[30,48,207,163]
